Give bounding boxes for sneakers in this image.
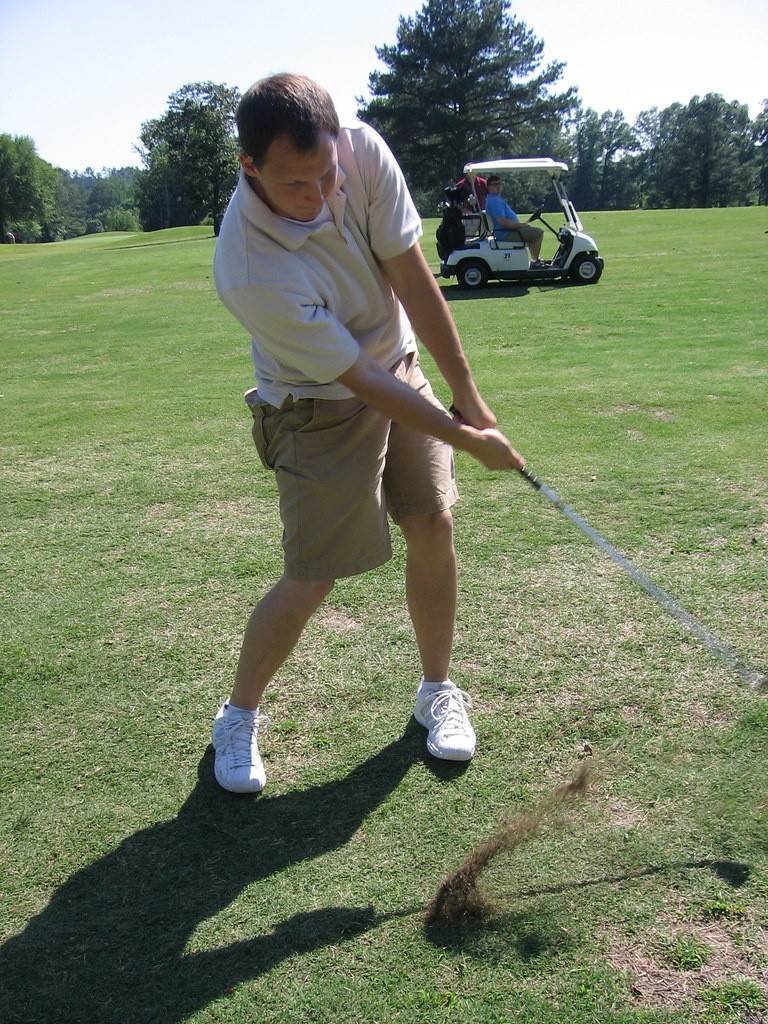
[211,700,266,794]
[413,674,477,761]
[529,257,550,270]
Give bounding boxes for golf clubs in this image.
[448,403,768,692]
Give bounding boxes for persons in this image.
[453,161,490,240]
[485,175,551,270]
[209,70,533,796]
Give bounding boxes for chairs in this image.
[481,210,530,250]
